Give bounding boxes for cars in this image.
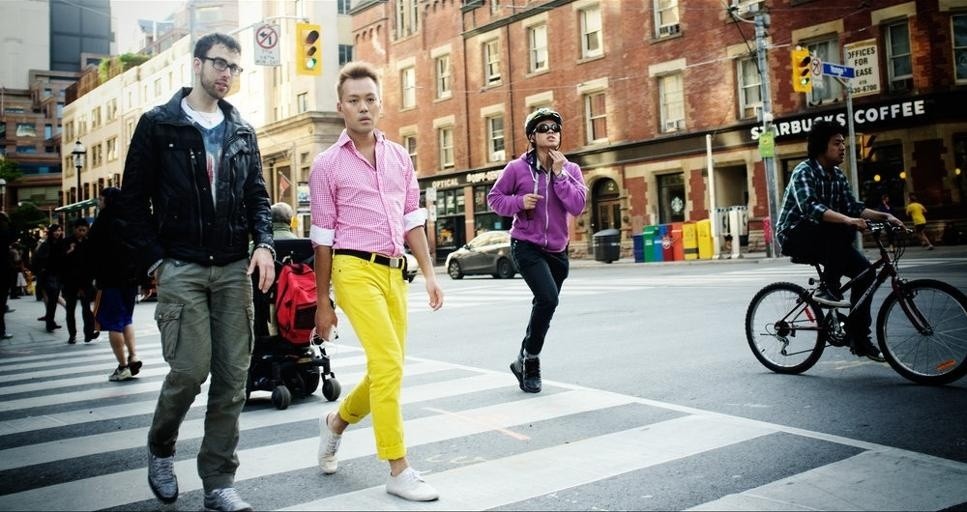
[445,230,519,280]
[403,248,419,283]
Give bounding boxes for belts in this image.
[330,249,406,270]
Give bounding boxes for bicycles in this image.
[745,218,967,385]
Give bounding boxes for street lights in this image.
[70,139,89,200]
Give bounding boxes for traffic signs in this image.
[822,63,854,77]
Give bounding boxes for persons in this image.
[487,108,586,394]
[121,32,276,512]
[905,192,935,251]
[248,202,299,261]
[879,194,896,250]
[776,119,908,359]
[1,187,157,382]
[308,62,445,502]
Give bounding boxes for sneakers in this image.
[146,439,180,502]
[317,410,344,474]
[522,357,541,393]
[204,487,252,510]
[858,337,885,362]
[509,353,521,383]
[386,466,439,501]
[812,280,852,308]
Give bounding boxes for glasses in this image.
[196,56,241,77]
[531,123,561,133]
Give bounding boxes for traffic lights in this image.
[297,23,321,75]
[791,49,812,92]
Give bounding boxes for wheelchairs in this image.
[248,236,341,408]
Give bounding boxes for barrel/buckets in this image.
[696,219,714,260]
[628,219,684,263]
[683,224,699,260]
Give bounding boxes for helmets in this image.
[525,108,564,139]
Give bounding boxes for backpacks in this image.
[274,254,316,344]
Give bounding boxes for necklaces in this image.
[186,99,219,126]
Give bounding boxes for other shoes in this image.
[109,360,142,380]
[85,332,100,342]
[3,334,13,339]
[47,325,62,332]
[37,316,46,321]
